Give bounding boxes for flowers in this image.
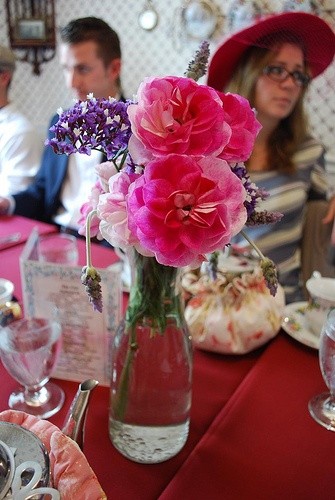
[47,35,281,428]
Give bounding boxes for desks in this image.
[0,213,335,499]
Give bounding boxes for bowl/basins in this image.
[0,277,14,304]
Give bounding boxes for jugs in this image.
[0,380,101,499]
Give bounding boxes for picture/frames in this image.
[16,17,48,41]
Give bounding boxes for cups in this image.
[283,302,329,350]
[37,234,78,267]
[305,271,335,334]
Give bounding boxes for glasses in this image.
[261,65,310,86]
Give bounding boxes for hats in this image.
[0,46,17,72]
[204,13,335,92]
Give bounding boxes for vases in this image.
[109,242,193,464]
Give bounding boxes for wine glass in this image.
[0,303,65,419]
[308,309,335,432]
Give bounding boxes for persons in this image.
[221,30,334,306]
[0,16,131,254]
[0,44,46,197]
